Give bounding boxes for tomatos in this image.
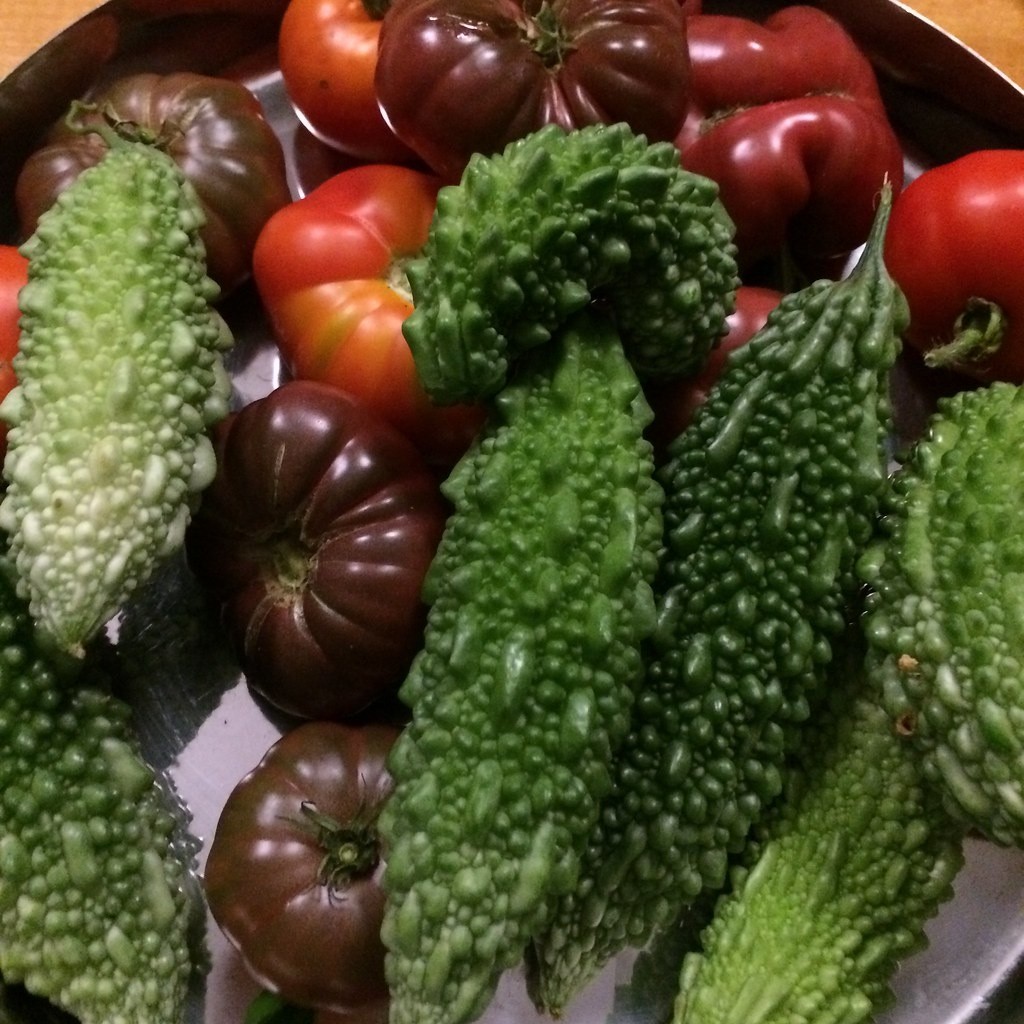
[0,0,1024,1014]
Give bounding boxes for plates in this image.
[1,5,1024,1023]
